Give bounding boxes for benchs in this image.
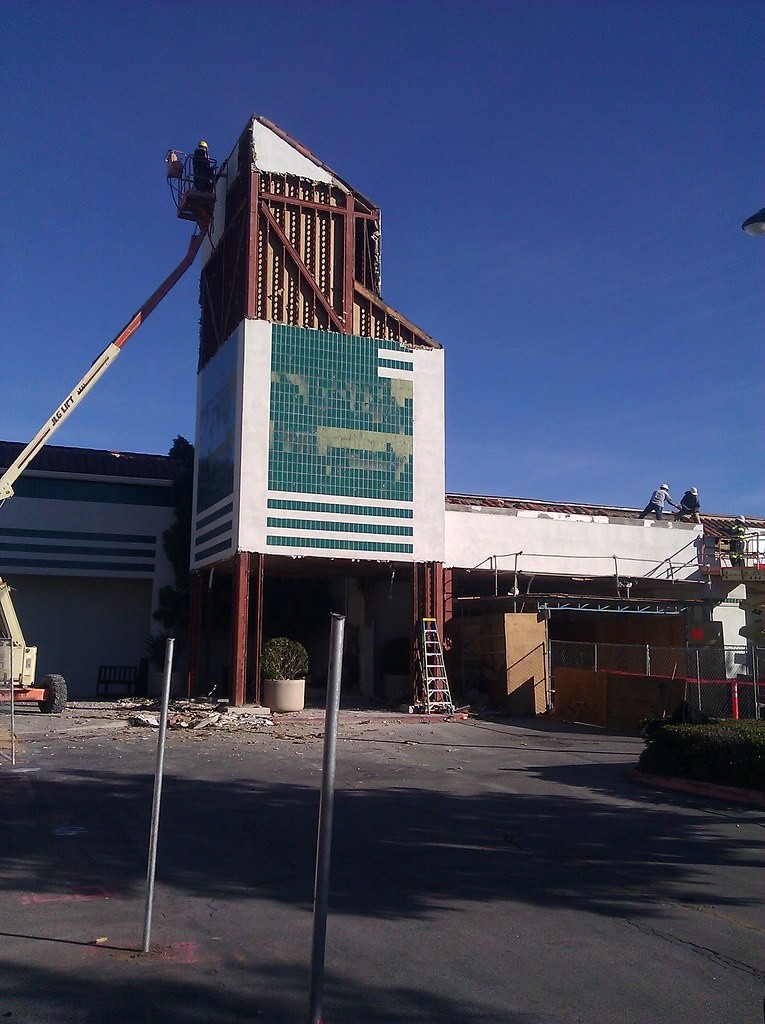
[96,664,140,696]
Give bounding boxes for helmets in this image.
[736,515,745,524]
[660,484,669,491]
[690,487,698,495]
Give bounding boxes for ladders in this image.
[422,617,455,716]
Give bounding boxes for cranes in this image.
[0,149,220,716]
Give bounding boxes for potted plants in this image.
[262,637,309,712]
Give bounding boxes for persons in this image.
[674,487,701,524]
[192,141,214,194]
[718,514,746,567]
[639,483,683,520]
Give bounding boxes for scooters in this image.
[638,699,697,750]
